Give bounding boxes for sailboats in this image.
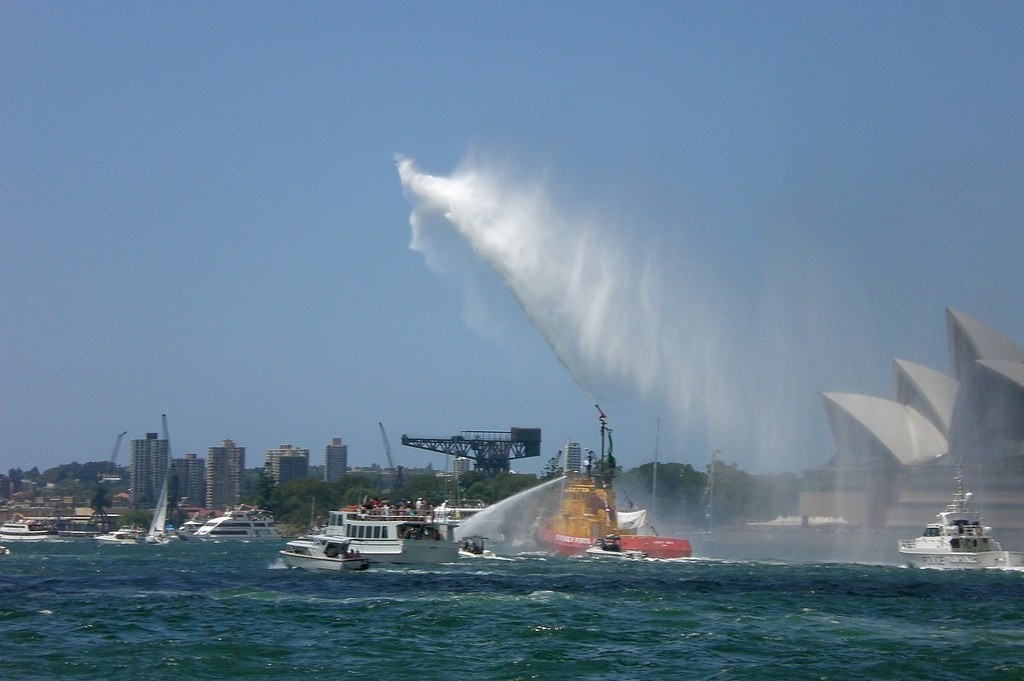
[144,478,172,545]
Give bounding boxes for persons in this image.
[358,493,477,558]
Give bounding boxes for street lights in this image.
[708,448,723,525]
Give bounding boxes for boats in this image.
[0,515,105,540]
[279,531,370,572]
[455,536,491,557]
[109,522,146,540]
[896,464,1024,571]
[175,504,284,540]
[297,509,461,564]
[528,404,694,562]
